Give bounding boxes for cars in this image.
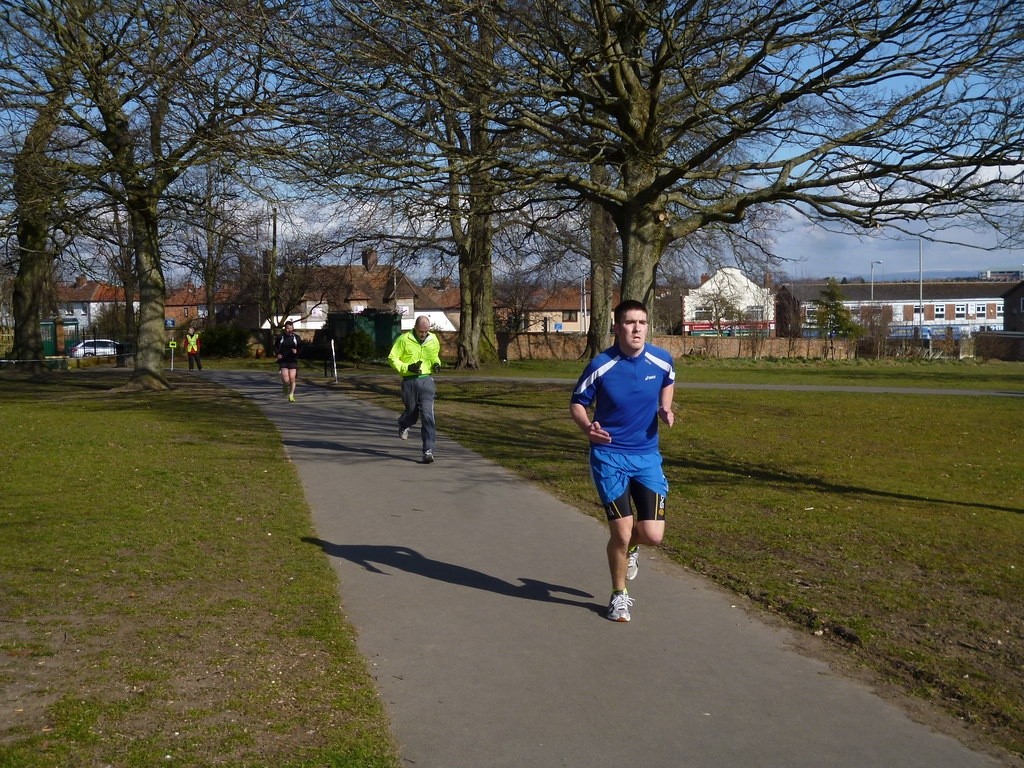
[68,340,120,359]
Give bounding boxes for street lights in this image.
[871,260,883,306]
[901,238,923,340]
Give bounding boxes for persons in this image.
[569,300,676,622]
[184,327,204,372]
[387,316,441,462]
[273,321,303,403]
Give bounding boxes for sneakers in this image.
[284,383,290,394]
[399,425,409,440]
[288,394,295,402]
[626,546,638,579]
[422,449,434,463]
[606,590,635,622]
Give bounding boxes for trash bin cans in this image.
[115,343,125,367]
